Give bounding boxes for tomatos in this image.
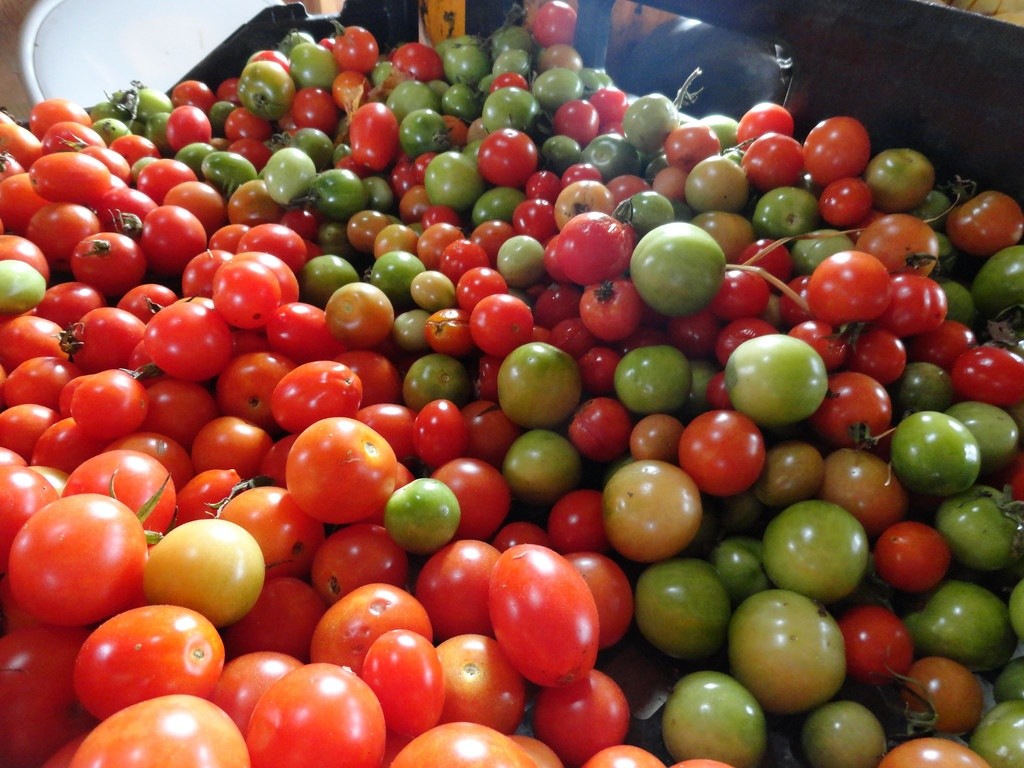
[0,0,1024,768]
